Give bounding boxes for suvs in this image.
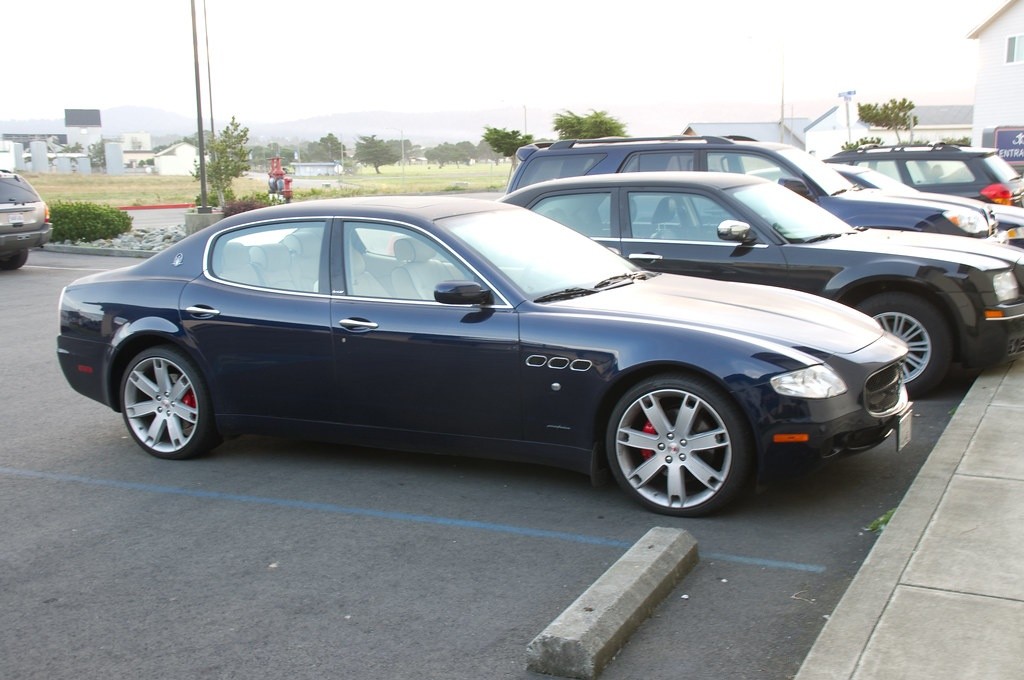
[504,134,1024,268]
[0,169,54,272]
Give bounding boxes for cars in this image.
[822,160,1024,250]
[56,197,914,515]
[451,170,1024,402]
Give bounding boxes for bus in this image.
[784,139,1024,210]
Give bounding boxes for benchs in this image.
[222,227,324,292]
[547,204,605,238]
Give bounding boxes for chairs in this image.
[652,197,678,239]
[390,239,456,302]
[350,248,390,297]
[629,199,640,239]
